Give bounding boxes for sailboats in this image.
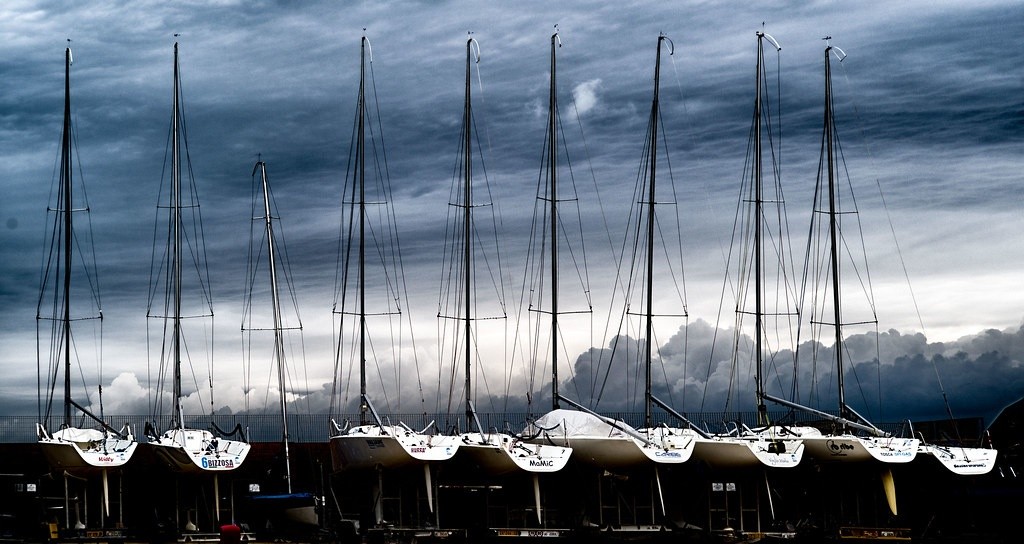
[790,44,999,477]
[698,31,920,466]
[149,44,251,472]
[588,32,805,470]
[329,36,460,477]
[229,158,329,528]
[438,37,572,479]
[504,32,696,468]
[34,37,138,472]
